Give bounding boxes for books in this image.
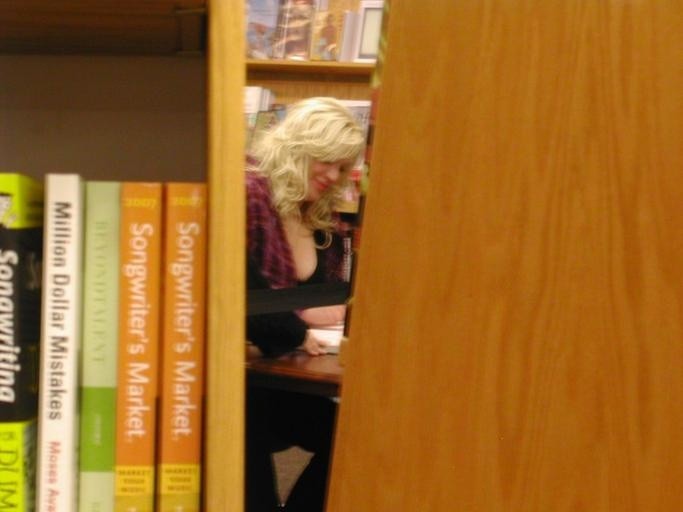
[246,0,383,62]
[245,86,372,167]
[296,322,345,355]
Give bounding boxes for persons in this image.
[247,242,329,357]
[245,98,366,511]
